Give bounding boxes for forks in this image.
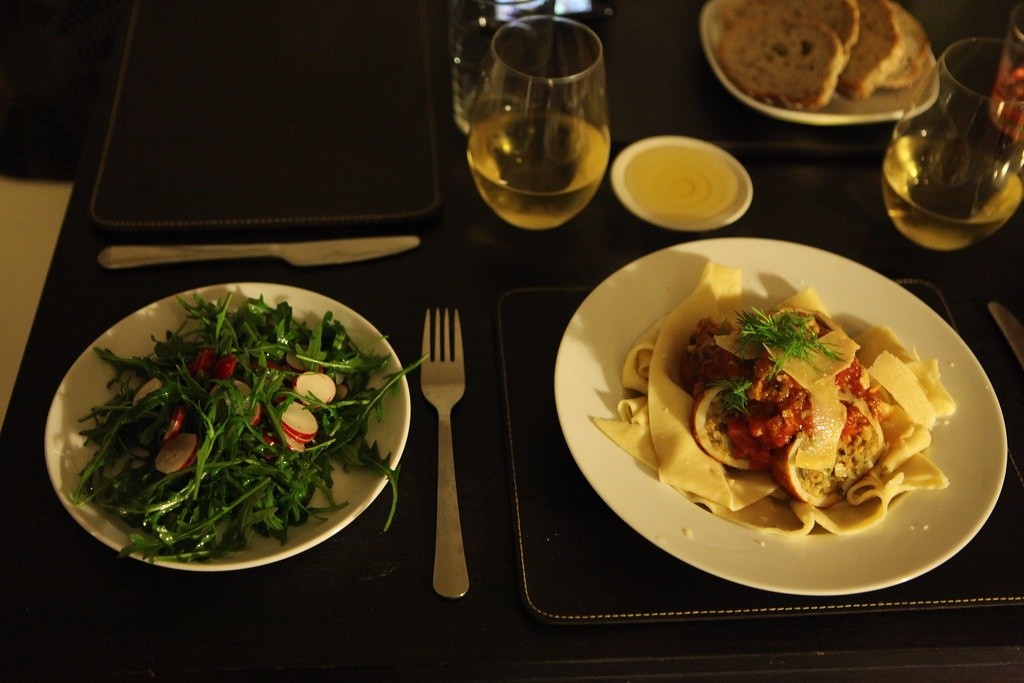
[420,308,469,598]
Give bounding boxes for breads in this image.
[713,0,930,110]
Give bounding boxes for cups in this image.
[451,14,610,229]
[448,0,556,135]
[881,39,1024,253]
[988,3,1024,141]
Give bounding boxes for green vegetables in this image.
[704,306,845,417]
[71,291,430,563]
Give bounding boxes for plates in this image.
[555,237,1008,598]
[611,135,753,230]
[44,282,411,572]
[701,0,940,126]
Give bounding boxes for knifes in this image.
[987,300,1024,366]
[97,235,420,267]
